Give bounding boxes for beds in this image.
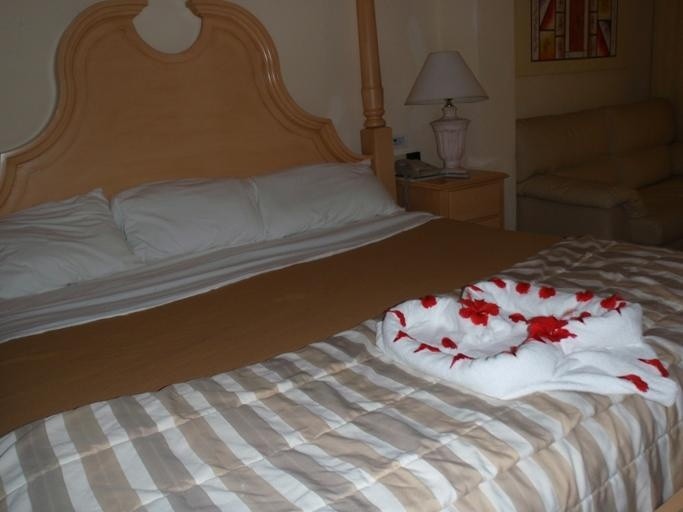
[1,0,683,512]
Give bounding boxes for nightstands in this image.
[394,166,510,233]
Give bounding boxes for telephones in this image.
[408,159,438,178]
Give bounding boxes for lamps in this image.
[401,49,490,176]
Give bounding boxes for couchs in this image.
[516,97,683,251]
